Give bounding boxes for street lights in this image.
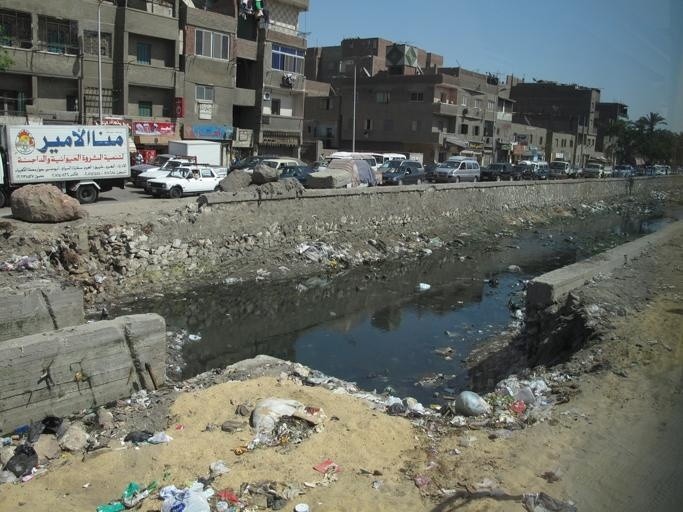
[352,64,371,152]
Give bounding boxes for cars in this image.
[146,165,225,197]
[279,165,316,188]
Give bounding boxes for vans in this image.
[228,155,304,175]
[242,158,300,177]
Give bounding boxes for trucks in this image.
[130,138,227,187]
[0,123,133,208]
[137,156,227,190]
